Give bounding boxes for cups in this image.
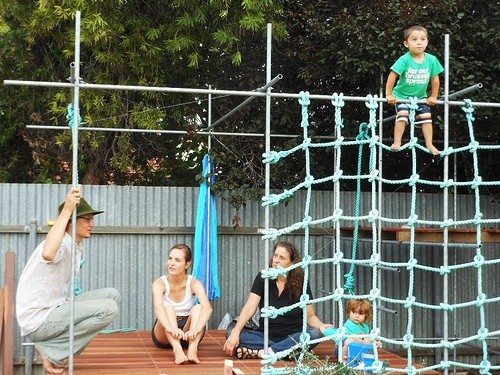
[364,354,375,372]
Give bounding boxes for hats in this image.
[59,197,104,220]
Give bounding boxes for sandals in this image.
[235,344,264,359]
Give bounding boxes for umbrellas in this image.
[192,153,221,331]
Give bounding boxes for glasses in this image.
[77,216,94,221]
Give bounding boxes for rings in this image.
[187,332,189,336]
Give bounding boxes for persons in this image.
[152,244,213,365]
[385,26,444,156]
[222,242,333,360]
[325,299,390,373]
[15,187,121,375]
